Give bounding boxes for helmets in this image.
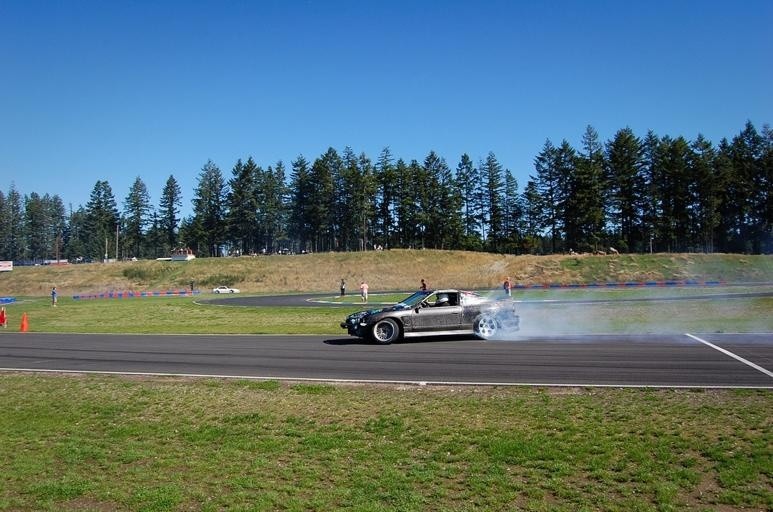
[435,294,449,304]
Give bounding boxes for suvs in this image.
[226,247,240,257]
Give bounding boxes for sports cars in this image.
[340,288,521,346]
[212,286,240,294]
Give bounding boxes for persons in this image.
[504,277,511,297]
[340,278,346,296]
[420,279,426,291]
[360,281,369,302]
[173,247,190,251]
[51,287,58,307]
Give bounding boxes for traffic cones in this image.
[0,306,7,325]
[19,311,28,332]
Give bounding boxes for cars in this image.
[253,246,271,255]
